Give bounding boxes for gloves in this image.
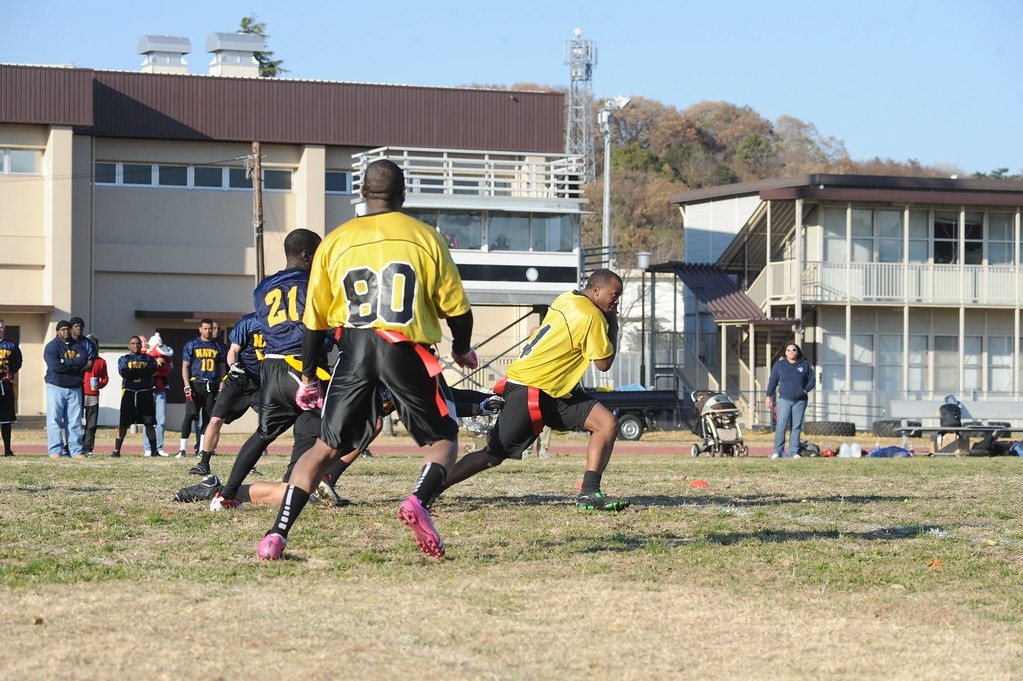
[451,346,478,372]
[295,379,323,409]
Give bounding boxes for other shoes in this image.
[145,450,151,457]
[87,451,96,459]
[175,452,185,458]
[151,451,160,457]
[111,451,119,457]
[198,451,203,457]
[159,449,169,456]
[5,450,14,456]
[773,454,780,459]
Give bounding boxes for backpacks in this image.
[970,438,991,455]
[939,403,961,427]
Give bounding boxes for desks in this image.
[895,424,1023,456]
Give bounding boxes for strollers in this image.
[689,388,748,458]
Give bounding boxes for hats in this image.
[69,317,84,327]
[56,320,71,331]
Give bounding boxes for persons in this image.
[83,334,109,456]
[444,232,460,248]
[524,326,552,458]
[175,319,227,458]
[188,312,260,475]
[0,319,22,457]
[766,343,816,458]
[211,228,383,513]
[257,159,478,562]
[171,403,394,507]
[139,333,173,456]
[110,336,161,457]
[491,235,509,249]
[44,317,94,458]
[427,269,630,518]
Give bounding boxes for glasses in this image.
[786,348,798,352]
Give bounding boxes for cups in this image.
[90,377,98,390]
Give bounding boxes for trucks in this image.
[580,390,678,441]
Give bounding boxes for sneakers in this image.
[257,530,285,558]
[188,464,210,475]
[209,492,244,512]
[173,474,221,503]
[575,490,630,512]
[312,477,349,506]
[397,495,445,558]
